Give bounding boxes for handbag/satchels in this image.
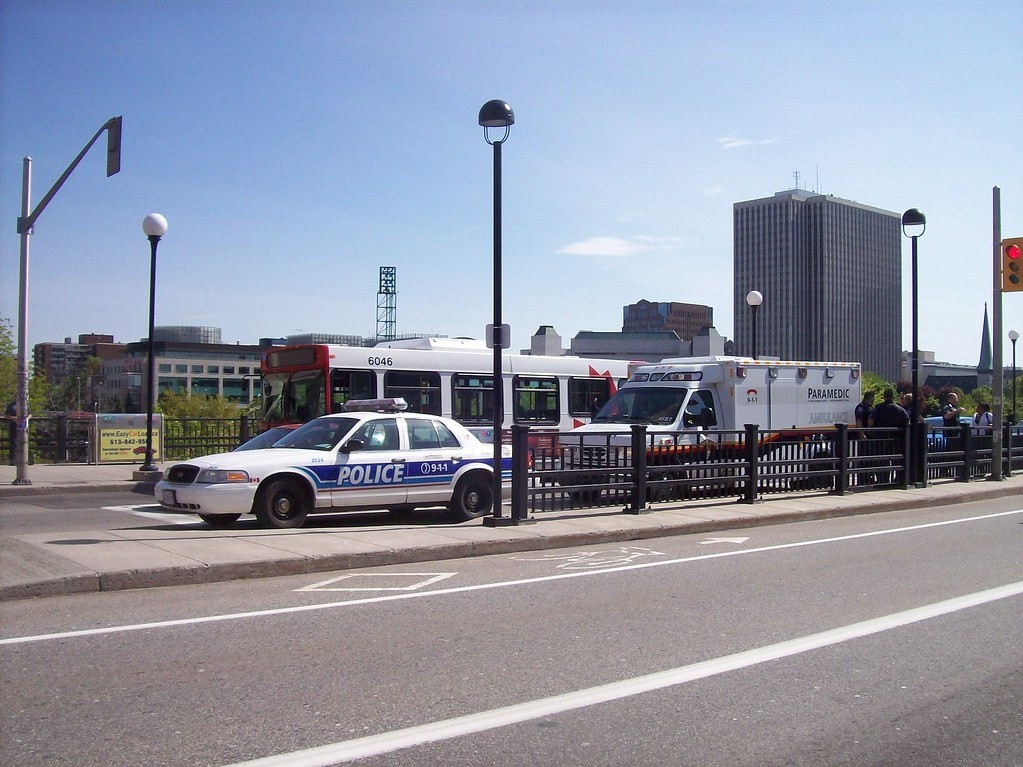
[985,412,993,436]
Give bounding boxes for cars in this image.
[153,396,541,529]
[923,416,974,452]
[1008,419,1023,447]
[233,423,303,449]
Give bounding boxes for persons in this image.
[5,392,34,466]
[897,391,925,424]
[971,402,993,435]
[1006,409,1013,422]
[855,392,874,485]
[868,390,910,483]
[942,392,965,437]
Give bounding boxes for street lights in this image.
[139,213,168,471]
[902,207,925,485]
[1008,329,1020,424]
[746,290,763,359]
[479,98,518,528]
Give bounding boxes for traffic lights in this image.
[1002,237,1023,292]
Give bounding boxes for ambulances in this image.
[553,352,862,506]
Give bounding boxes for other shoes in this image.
[939,473,953,478]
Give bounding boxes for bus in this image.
[240,337,648,483]
